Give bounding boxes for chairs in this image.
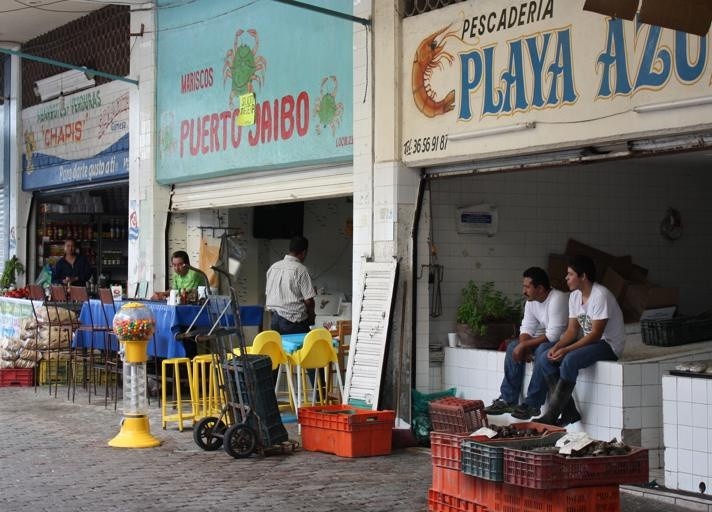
[287,329,338,413]
[323,320,351,407]
[232,329,295,417]
[28,283,60,394]
[49,283,84,399]
[96,286,160,413]
[63,283,112,403]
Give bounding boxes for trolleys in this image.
[185,266,292,452]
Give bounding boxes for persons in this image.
[533,258,625,427]
[51,237,92,287]
[171,251,212,307]
[483,267,569,419]
[265,235,326,402]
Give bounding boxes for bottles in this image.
[177,287,198,305]
[47,218,128,299]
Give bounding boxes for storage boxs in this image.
[70,356,101,387]
[100,358,124,384]
[38,360,71,386]
[0,369,33,386]
[298,403,396,458]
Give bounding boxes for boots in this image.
[545,374,581,427]
[531,378,576,425]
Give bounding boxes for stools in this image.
[161,358,196,431]
[192,353,231,425]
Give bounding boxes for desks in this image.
[72,299,263,409]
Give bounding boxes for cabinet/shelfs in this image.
[41,213,99,294]
[96,215,128,292]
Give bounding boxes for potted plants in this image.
[455,280,526,349]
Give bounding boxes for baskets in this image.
[427,396,649,512]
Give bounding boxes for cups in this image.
[448,333,458,348]
